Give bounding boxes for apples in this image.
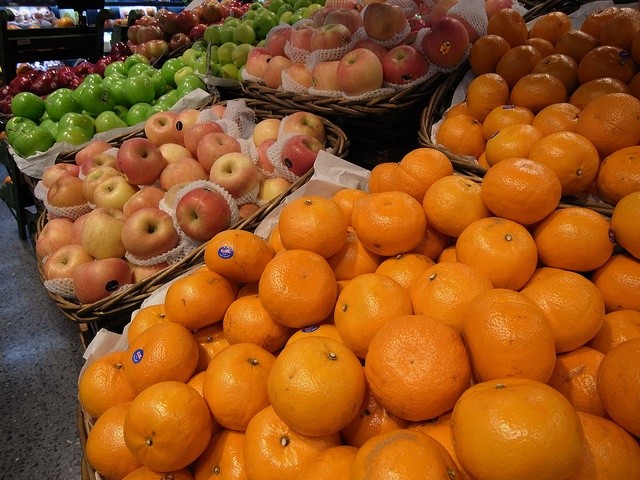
[0,58,327,304]
[115,2,515,99]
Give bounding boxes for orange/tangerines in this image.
[348,0,640,197]
[78,198,640,480]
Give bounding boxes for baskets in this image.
[5,86,221,214]
[239,69,450,150]
[418,0,615,222]
[31,98,354,322]
